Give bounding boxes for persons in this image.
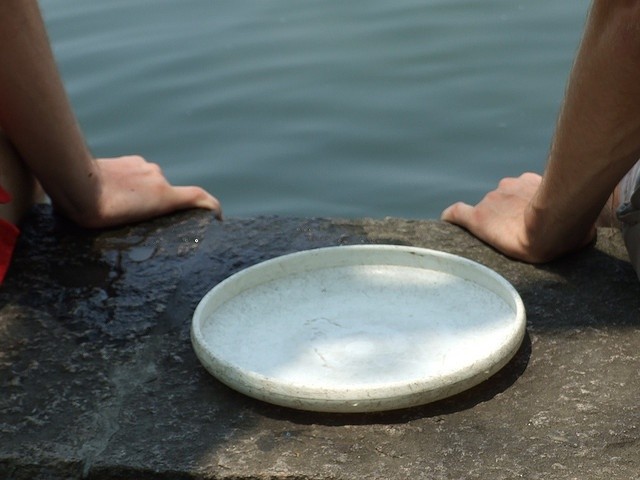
[0,0,223,285]
[439,0,640,283]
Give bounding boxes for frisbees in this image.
[191,244,527,413]
